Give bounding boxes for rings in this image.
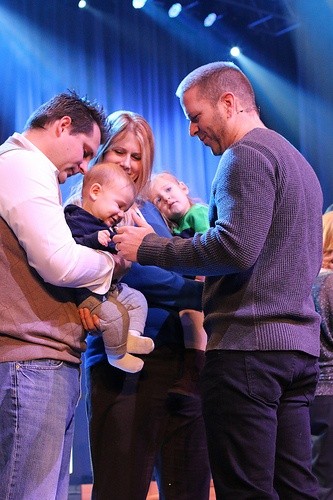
[115,243,120,251]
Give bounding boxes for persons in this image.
[112,62,325,500]
[0,92,333,500]
